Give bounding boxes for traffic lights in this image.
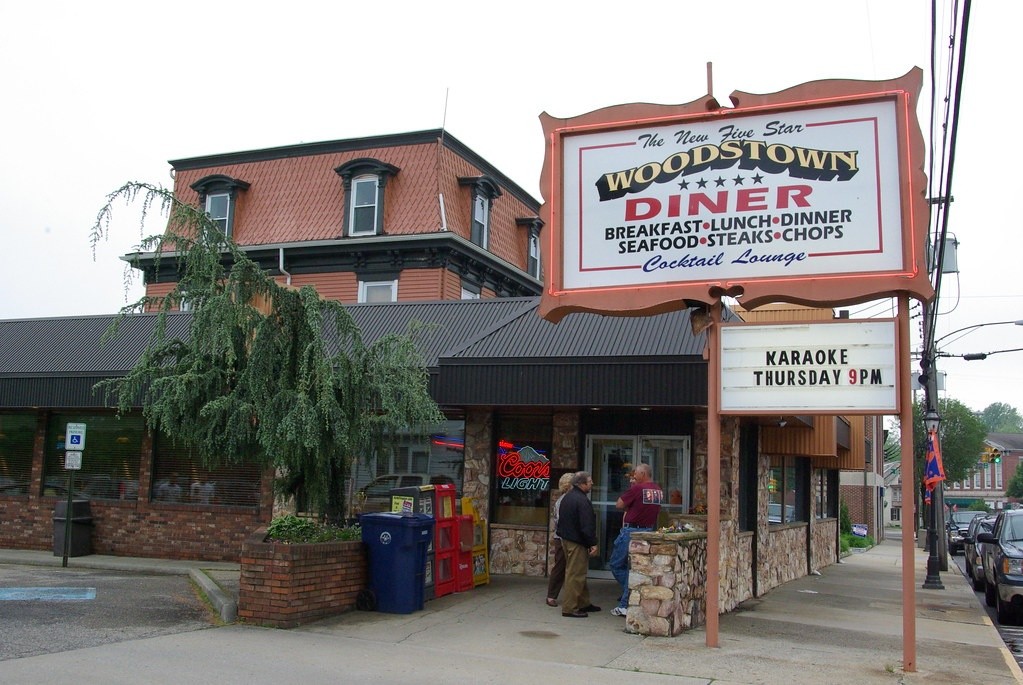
[988,449,1002,463]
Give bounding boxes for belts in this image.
[623,524,653,529]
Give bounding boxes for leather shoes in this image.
[580,604,601,612]
[562,610,588,618]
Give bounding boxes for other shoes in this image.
[610,607,627,617]
[546,598,558,607]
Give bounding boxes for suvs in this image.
[946,510,988,556]
[976,507,1022,625]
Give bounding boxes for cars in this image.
[964,515,996,590]
[959,512,997,577]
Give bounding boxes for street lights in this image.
[922,406,946,589]
[920,319,1022,573]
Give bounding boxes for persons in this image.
[610,464,663,617]
[545,471,601,617]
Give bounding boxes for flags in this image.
[923,428,946,506]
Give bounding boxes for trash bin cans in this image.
[917,529,928,549]
[53,499,94,558]
[390,483,436,602]
[356,510,437,615]
